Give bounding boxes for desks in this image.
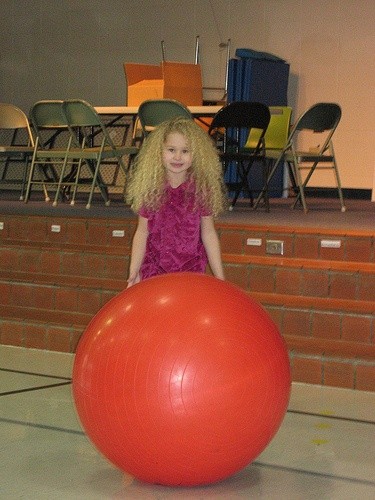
[61,106,223,203]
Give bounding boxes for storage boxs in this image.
[123,62,203,106]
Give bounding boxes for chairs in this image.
[61,98,139,210]
[139,99,193,137]
[253,103,346,213]
[25,100,111,209]
[207,101,271,212]
[0,103,50,203]
[234,106,303,208]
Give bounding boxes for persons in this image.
[123,117,229,289]
